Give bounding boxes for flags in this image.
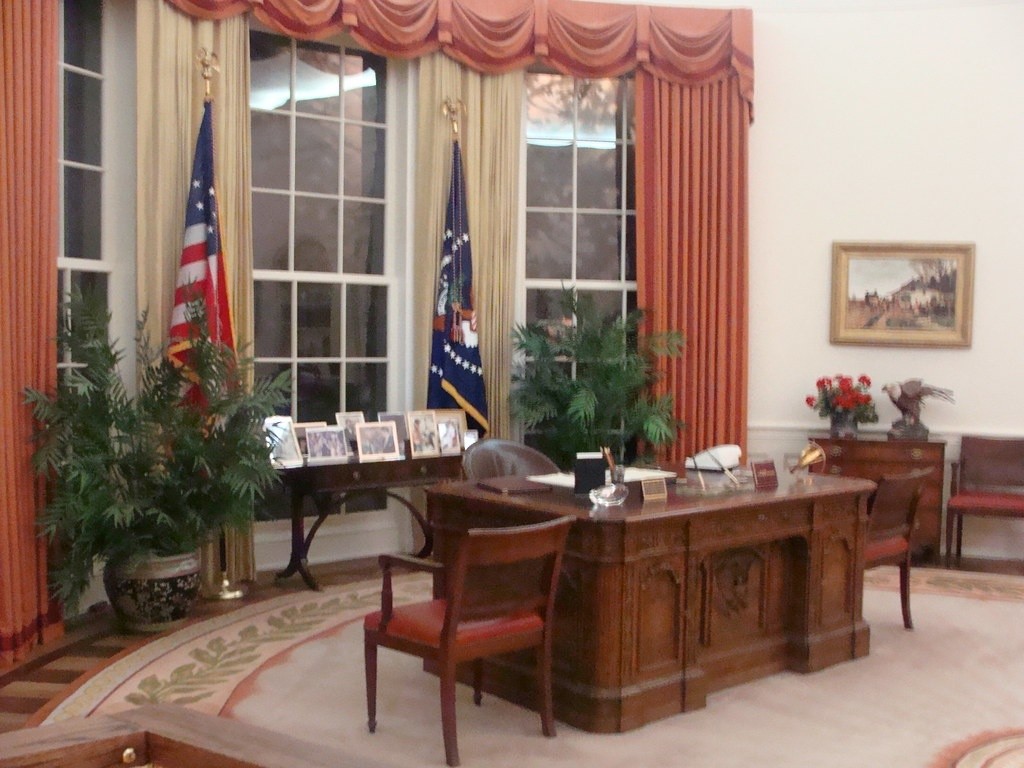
[426,141,488,438]
[167,94,242,439]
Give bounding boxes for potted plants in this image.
[17,267,292,635]
[503,276,690,484]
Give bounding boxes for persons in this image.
[443,421,458,448]
[363,427,395,455]
[310,432,341,457]
[412,419,435,452]
[345,419,356,440]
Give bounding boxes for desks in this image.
[274,456,463,591]
[424,458,878,736]
[806,435,946,565]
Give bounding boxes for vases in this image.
[827,412,858,440]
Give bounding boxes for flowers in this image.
[805,373,878,426]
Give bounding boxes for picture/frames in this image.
[831,240,974,350]
[265,406,470,469]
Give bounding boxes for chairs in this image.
[861,467,934,631]
[364,516,575,768]
[942,436,1023,573]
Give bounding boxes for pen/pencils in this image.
[599,445,615,471]
